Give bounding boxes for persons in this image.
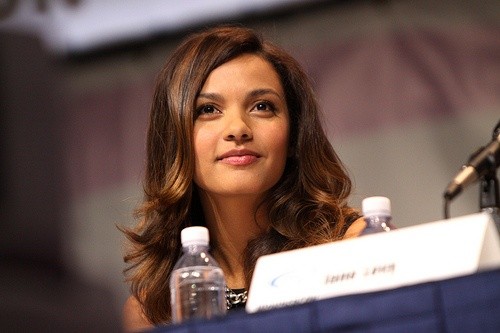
[113,27,394,333]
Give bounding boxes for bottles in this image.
[170,226,227,324]
[357,195,397,237]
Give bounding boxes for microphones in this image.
[444,121,500,202]
